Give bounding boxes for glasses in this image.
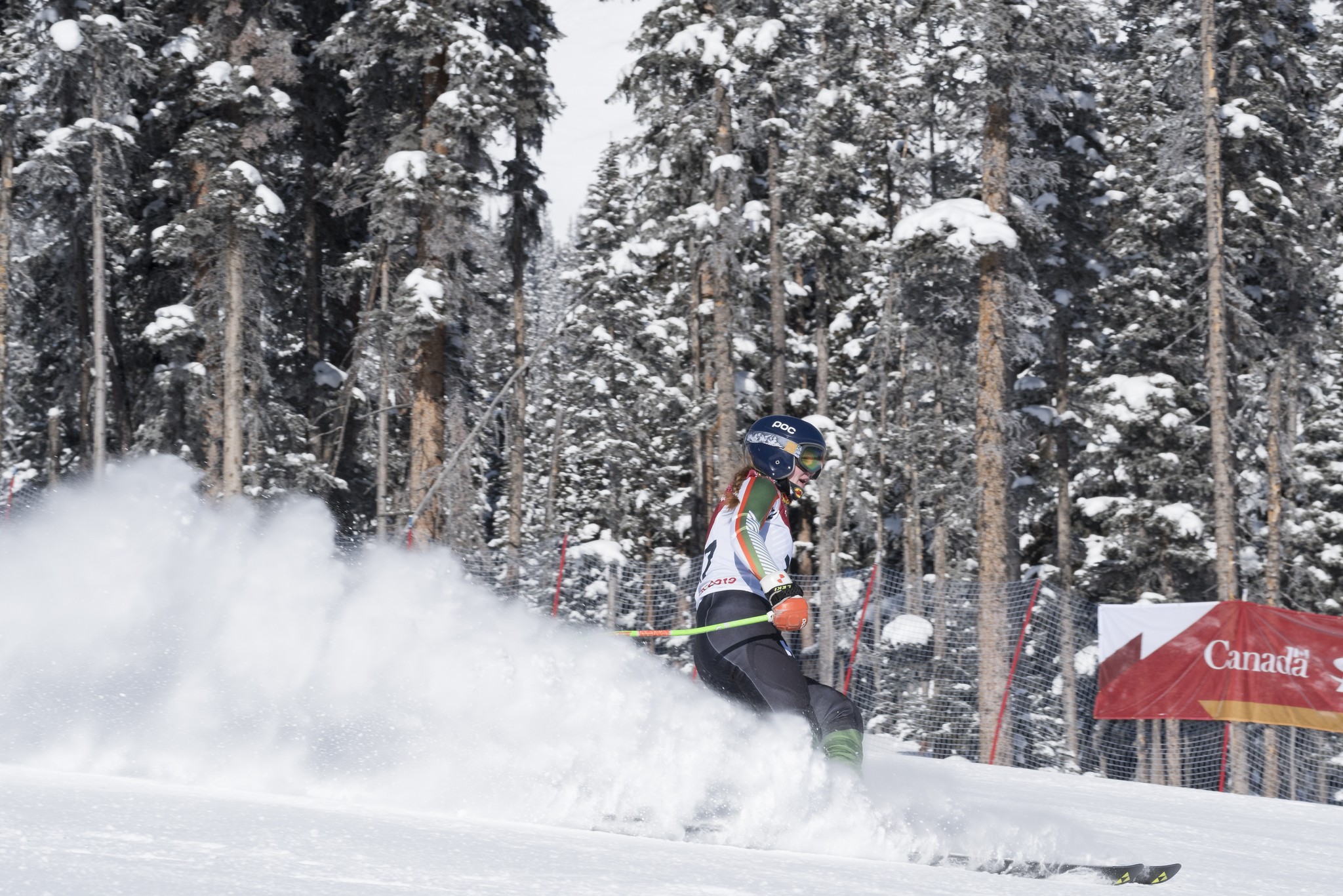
[797,444,824,480]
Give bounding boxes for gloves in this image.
[758,569,809,631]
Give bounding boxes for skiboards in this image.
[546,800,1181,888]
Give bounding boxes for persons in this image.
[691,415,864,773]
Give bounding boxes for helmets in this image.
[742,415,826,479]
